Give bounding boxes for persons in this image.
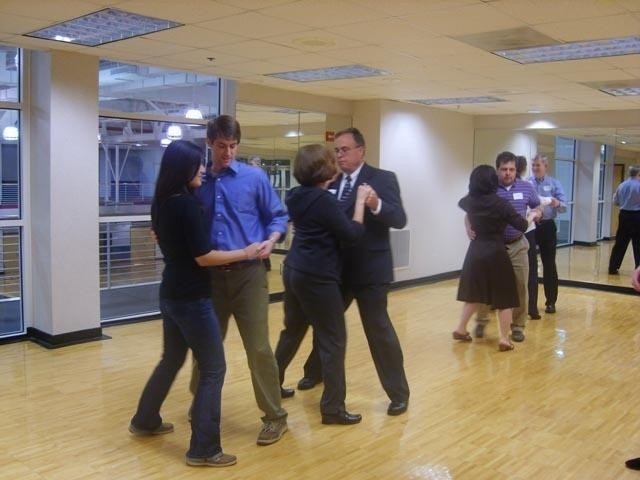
[247,156,261,166]
[287,128,410,416]
[465,151,543,342]
[515,156,552,320]
[149,115,291,446]
[128,139,266,468]
[273,144,372,424]
[452,164,538,352]
[608,165,640,274]
[631,266,640,291]
[526,154,568,313]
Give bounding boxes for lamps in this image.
[2,108,20,141]
[158,71,204,149]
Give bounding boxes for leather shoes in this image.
[453,331,472,341]
[297,377,323,389]
[323,412,362,425]
[280,387,294,397]
[499,343,514,351]
[387,402,407,416]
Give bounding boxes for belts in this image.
[220,263,231,271]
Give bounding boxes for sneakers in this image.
[476,325,484,337]
[127,421,174,435]
[545,305,555,313]
[257,421,288,446]
[512,331,524,342]
[528,313,541,319]
[186,454,236,467]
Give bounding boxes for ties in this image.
[340,176,351,199]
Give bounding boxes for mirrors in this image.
[473,127,640,299]
[233,98,329,297]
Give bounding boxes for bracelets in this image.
[244,248,248,261]
[270,238,275,246]
